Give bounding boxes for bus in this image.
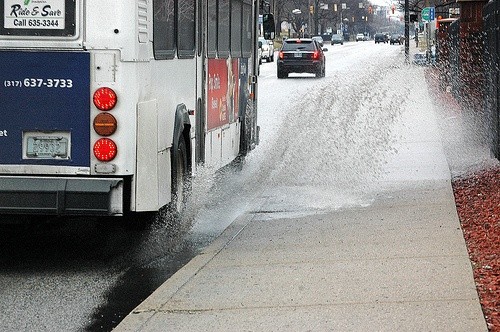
[0,0,277,220]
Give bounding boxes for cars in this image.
[330,34,344,46]
[390,34,404,45]
[258,36,275,65]
[277,38,329,79]
[312,36,324,47]
[356,34,365,42]
[374,33,389,44]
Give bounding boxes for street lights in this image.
[341,8,350,35]
